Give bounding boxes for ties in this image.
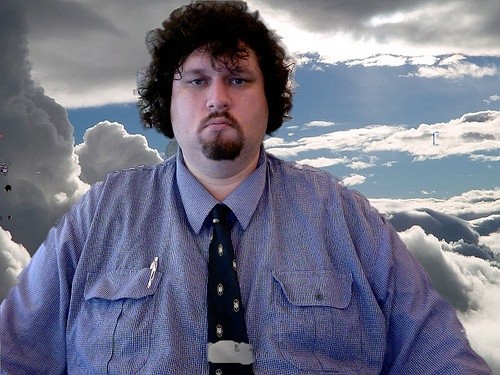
[204,203,255,375]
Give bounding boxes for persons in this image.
[1,0,495,371]
